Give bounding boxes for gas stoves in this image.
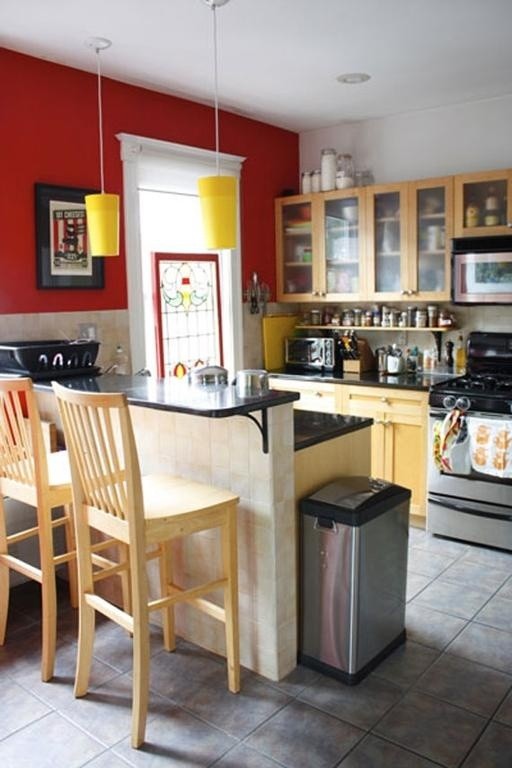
[427,370,511,414]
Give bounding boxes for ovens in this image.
[427,408,511,551]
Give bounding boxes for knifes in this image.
[337,330,359,359]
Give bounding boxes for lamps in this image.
[192,0,243,252]
[84,37,124,259]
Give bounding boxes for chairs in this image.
[1,375,137,685]
[48,379,242,753]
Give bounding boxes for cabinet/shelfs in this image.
[453,170,511,236]
[370,175,452,300]
[337,384,429,530]
[277,187,368,302]
[268,376,337,414]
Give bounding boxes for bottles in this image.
[310,305,450,328]
[300,144,374,196]
[376,333,467,374]
[111,344,129,375]
[484,186,499,210]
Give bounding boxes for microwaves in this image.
[283,336,340,371]
[454,253,511,304]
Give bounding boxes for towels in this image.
[468,415,512,477]
[430,409,468,475]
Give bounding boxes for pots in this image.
[236,368,268,389]
[187,367,228,382]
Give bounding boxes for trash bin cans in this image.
[298,476,412,685]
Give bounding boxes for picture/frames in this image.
[151,250,223,383]
[31,180,106,292]
[448,252,512,307]
